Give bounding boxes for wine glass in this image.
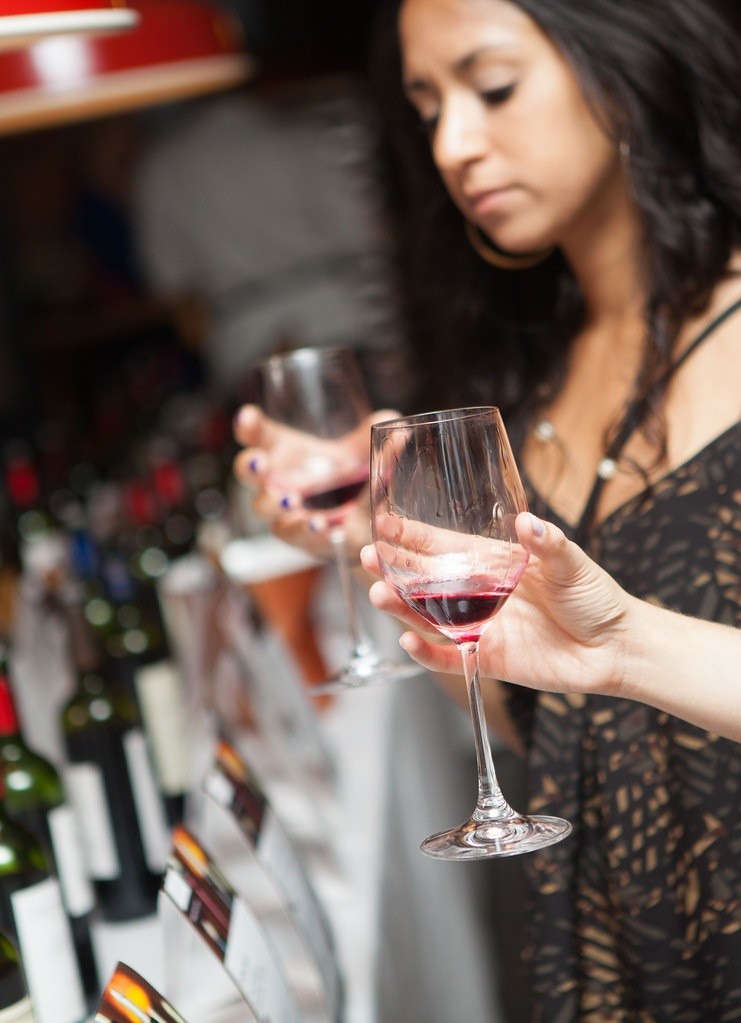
[369,406,572,861]
[259,344,424,696]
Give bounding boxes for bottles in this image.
[0,465,210,1023]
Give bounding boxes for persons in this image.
[233,0,741,1023]
